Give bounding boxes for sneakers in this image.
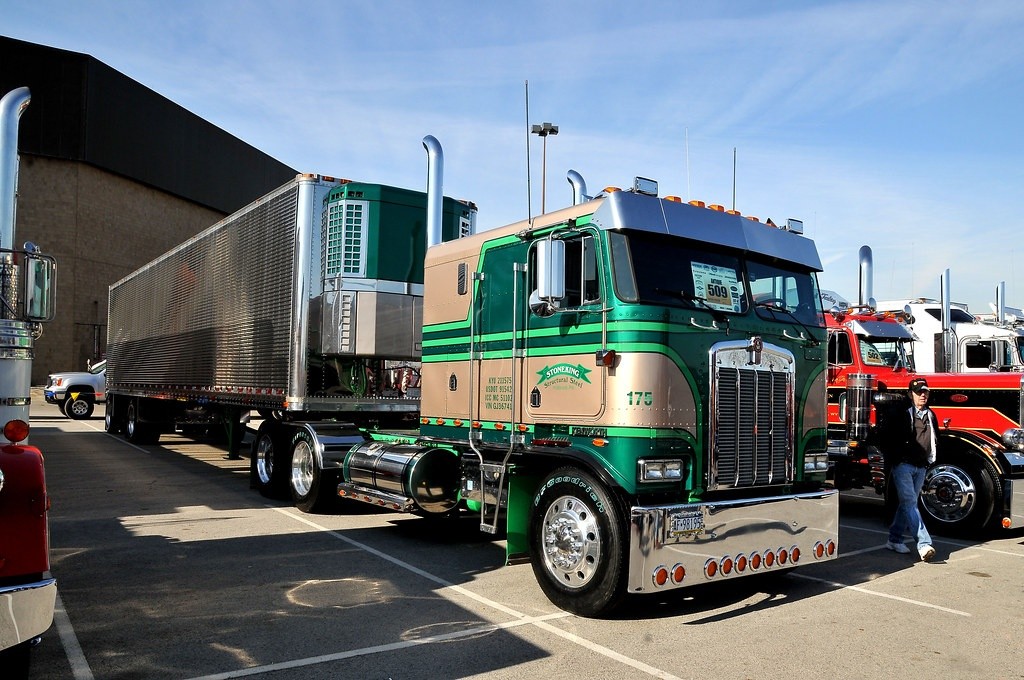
[918,544,935,561]
[885,540,910,553]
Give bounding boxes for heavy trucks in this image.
[0,84,60,658]
[102,132,841,620]
[750,244,1024,539]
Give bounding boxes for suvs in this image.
[44,359,106,419]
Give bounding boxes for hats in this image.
[909,378,930,393]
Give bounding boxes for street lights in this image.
[529,122,559,214]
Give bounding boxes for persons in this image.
[879,377,943,562]
